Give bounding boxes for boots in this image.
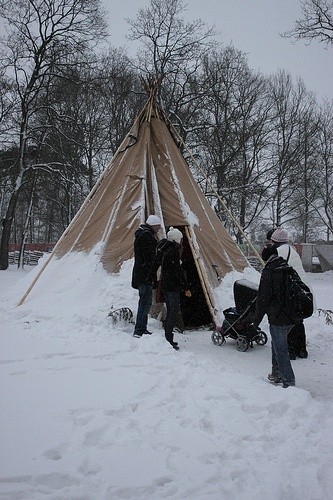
[165,333,179,349]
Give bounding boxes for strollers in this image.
[211,278,268,351]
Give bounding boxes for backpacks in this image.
[266,265,313,321]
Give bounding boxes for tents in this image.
[17,70,266,334]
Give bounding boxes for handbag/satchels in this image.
[156,275,165,303]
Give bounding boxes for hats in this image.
[267,228,276,240]
[167,227,182,244]
[262,244,278,260]
[271,228,289,242]
[147,215,161,225]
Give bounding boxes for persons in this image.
[155,226,191,352]
[257,243,297,389]
[131,215,162,339]
[265,228,318,361]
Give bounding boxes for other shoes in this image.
[289,351,307,360]
[268,374,295,388]
[134,330,152,338]
